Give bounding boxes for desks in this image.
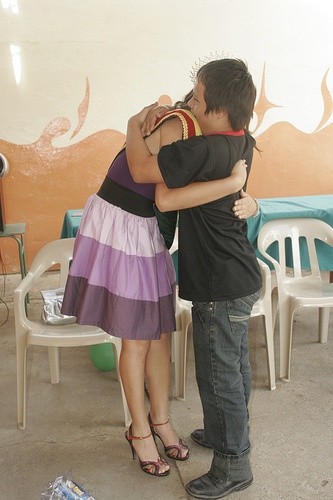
[0,223,29,318]
[60,194,333,284]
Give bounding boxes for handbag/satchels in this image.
[40,287,77,326]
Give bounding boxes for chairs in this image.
[169,227,276,400]
[257,218,333,382]
[14,238,133,430]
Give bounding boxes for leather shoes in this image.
[185,471,254,500]
[190,429,214,449]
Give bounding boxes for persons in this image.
[61,89,262,477]
[126,58,263,500]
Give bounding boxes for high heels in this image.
[124,423,172,476]
[147,411,189,461]
